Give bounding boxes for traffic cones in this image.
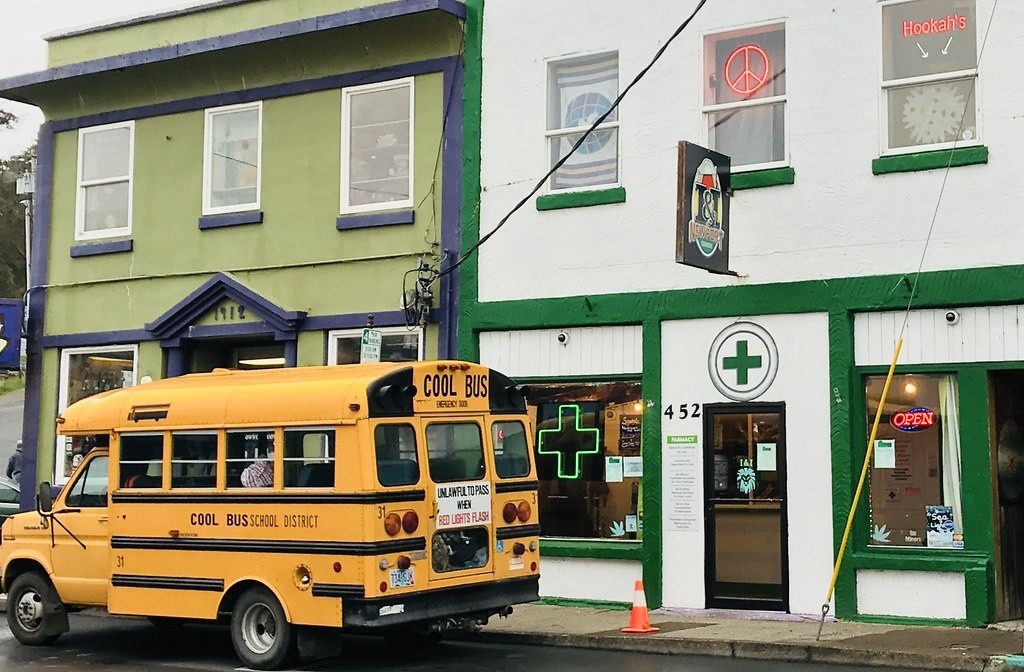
[621,578,662,634]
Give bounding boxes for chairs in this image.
[281,453,530,489]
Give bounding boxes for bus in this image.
[0,359,545,671]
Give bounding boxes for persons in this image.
[7,440,24,502]
